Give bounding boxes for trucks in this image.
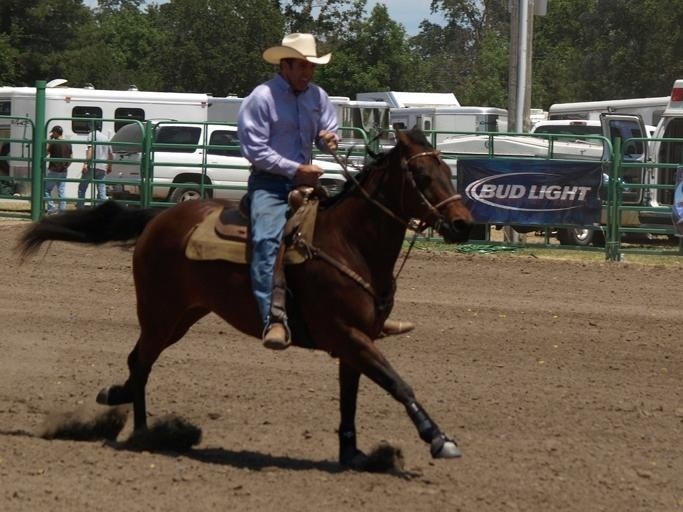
[597,76,682,230]
[525,118,656,246]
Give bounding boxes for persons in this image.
[76,121,113,209]
[44,125,72,215]
[236,34,415,348]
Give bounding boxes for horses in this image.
[3,112,478,470]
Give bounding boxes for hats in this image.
[262,31,334,68]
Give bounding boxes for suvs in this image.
[106,117,361,201]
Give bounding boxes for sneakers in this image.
[260,317,291,349]
[381,316,414,337]
